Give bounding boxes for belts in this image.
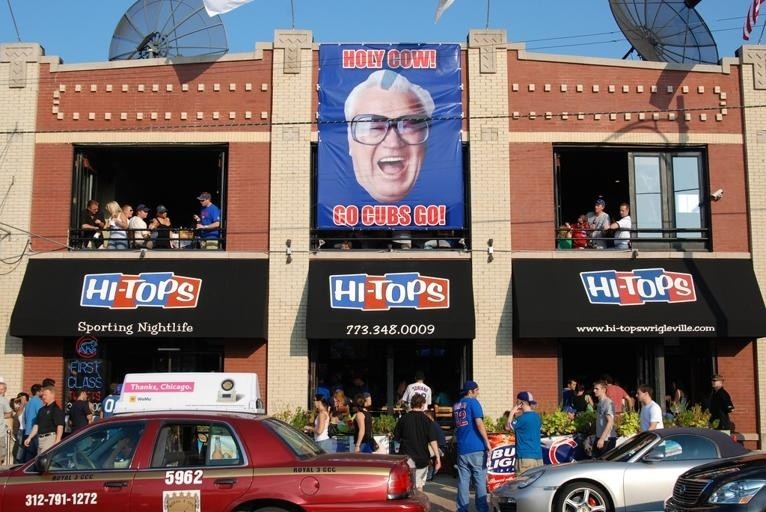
[40,433,51,437]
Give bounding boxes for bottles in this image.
[212,436,223,459]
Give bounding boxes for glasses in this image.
[576,221,582,225]
[351,113,432,146]
[201,200,204,202]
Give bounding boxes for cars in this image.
[667,451,764,512]
[485,428,753,511]
[2,373,431,510]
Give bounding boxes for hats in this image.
[595,199,605,204]
[196,193,211,201]
[136,204,151,212]
[156,205,168,213]
[460,380,478,389]
[517,391,537,405]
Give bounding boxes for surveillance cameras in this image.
[712,189,724,200]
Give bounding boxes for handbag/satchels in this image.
[328,423,339,438]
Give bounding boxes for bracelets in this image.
[204,224,208,229]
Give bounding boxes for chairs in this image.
[162,451,186,468]
[434,404,453,430]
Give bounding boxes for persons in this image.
[192,191,221,249]
[305,373,493,511]
[565,215,594,248]
[585,199,610,248]
[148,206,172,248]
[505,391,543,476]
[609,201,632,250]
[123,205,133,221]
[0,377,122,470]
[101,430,140,469]
[80,199,105,248]
[562,374,735,466]
[128,204,150,247]
[559,223,572,248]
[104,201,127,248]
[343,69,435,203]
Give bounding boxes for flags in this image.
[742,0,764,39]
